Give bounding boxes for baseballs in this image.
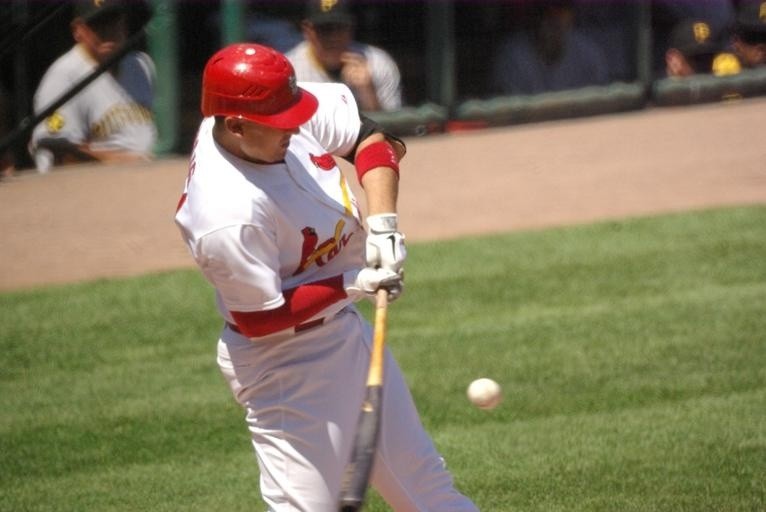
[712,51,740,77]
[466,377,501,409]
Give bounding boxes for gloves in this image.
[343,213,407,302]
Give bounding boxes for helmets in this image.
[200,43,319,129]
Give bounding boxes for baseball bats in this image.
[338,261,388,510]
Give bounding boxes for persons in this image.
[28,0,161,173]
[664,0,766,100]
[174,40,483,511]
[479,3,626,95]
[278,0,406,113]
